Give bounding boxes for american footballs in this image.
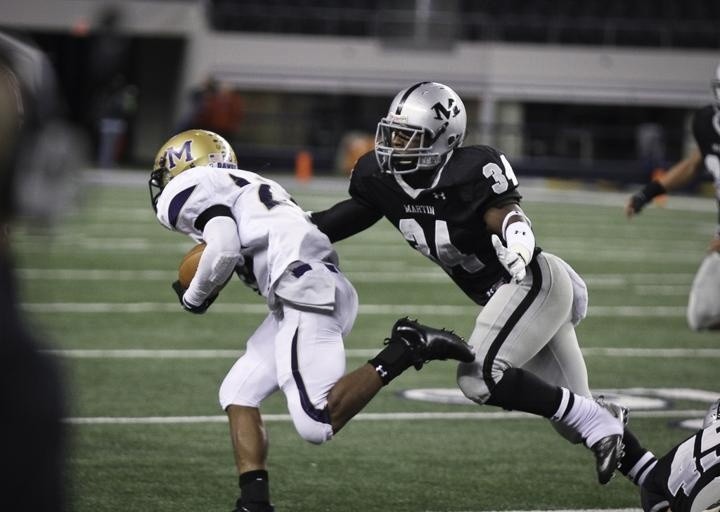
[178,242,237,299]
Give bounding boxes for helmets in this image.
[148,130,238,214]
[374,83,467,174]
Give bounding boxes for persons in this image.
[622,58,720,333]
[304,78,661,490]
[641,398,720,512]
[149,129,475,512]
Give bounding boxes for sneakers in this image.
[383,316,475,370]
[585,395,629,485]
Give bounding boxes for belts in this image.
[292,264,336,278]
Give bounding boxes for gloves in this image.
[492,235,527,283]
[172,280,219,314]
